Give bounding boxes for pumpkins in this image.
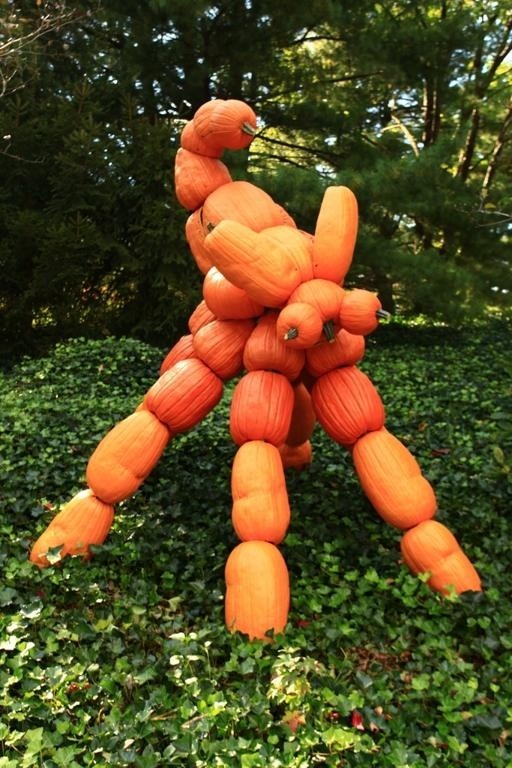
[29,99,483,644]
